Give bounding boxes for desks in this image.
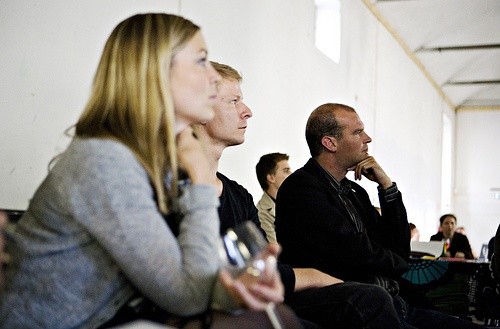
[397,258,493,320]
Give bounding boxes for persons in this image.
[1,13,284,327]
[488,224,500,285]
[275,103,495,329]
[255,152,292,246]
[408,222,417,239]
[430,214,475,259]
[163,58,399,329]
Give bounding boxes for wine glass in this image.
[442,238,449,253]
[216,221,287,329]
